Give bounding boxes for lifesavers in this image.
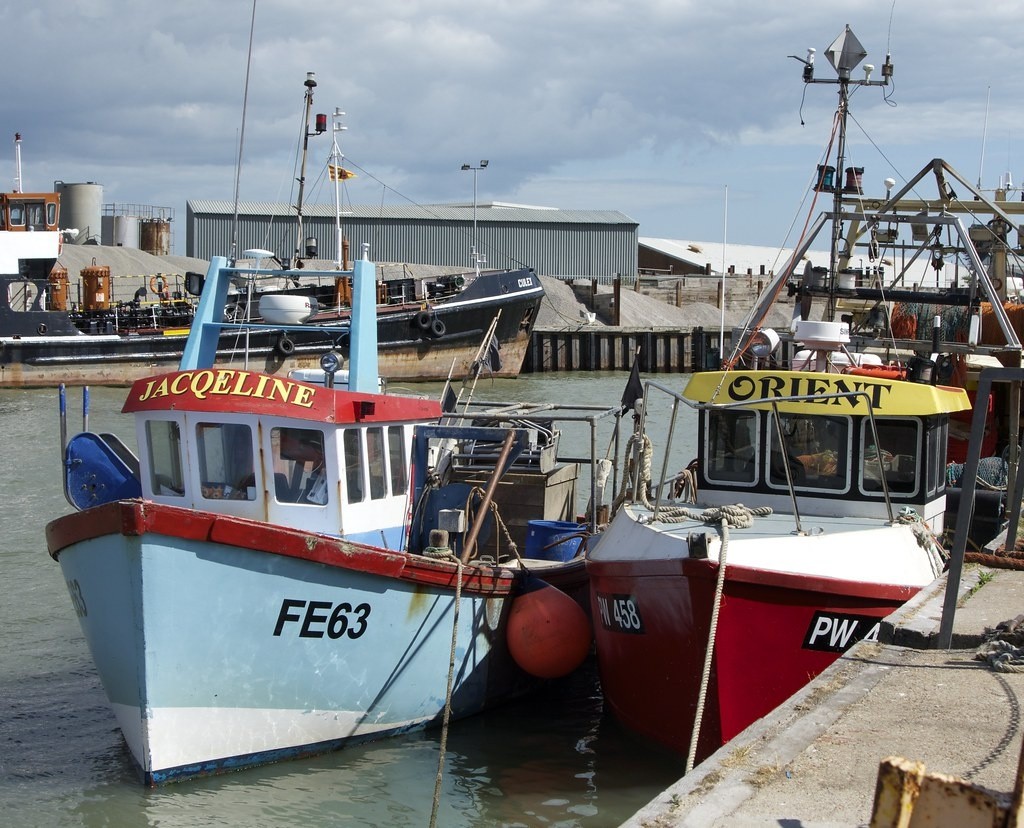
[841,363,907,380]
[149,276,168,295]
[415,311,431,330]
[277,338,296,357]
[430,319,446,337]
[455,277,466,288]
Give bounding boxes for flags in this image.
[328,164,358,181]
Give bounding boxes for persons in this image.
[298,429,328,506]
[725,415,753,473]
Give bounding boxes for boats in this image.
[0,71,549,394]
[580,22,1023,770]
[41,67,654,783]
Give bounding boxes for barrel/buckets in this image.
[525,521,586,563]
